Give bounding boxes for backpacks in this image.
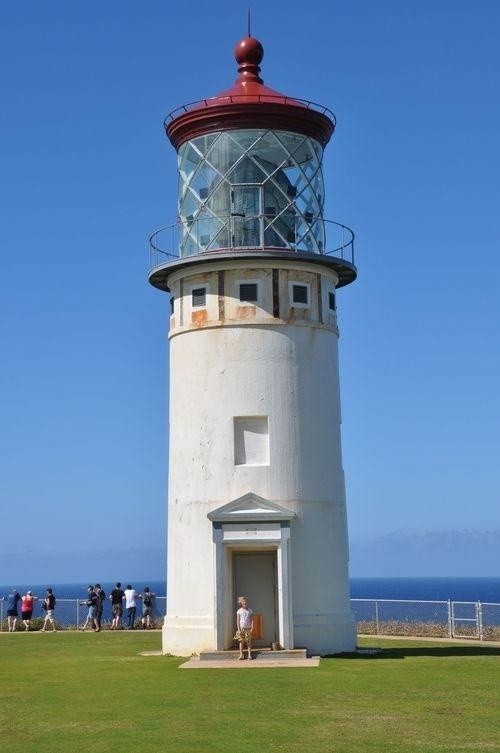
[143,594,152,607]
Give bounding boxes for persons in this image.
[20,590,39,630]
[123,584,138,629]
[78,584,101,632]
[39,587,57,632]
[107,581,126,630]
[92,584,107,630]
[1,589,22,631]
[139,585,158,628]
[230,595,256,660]
